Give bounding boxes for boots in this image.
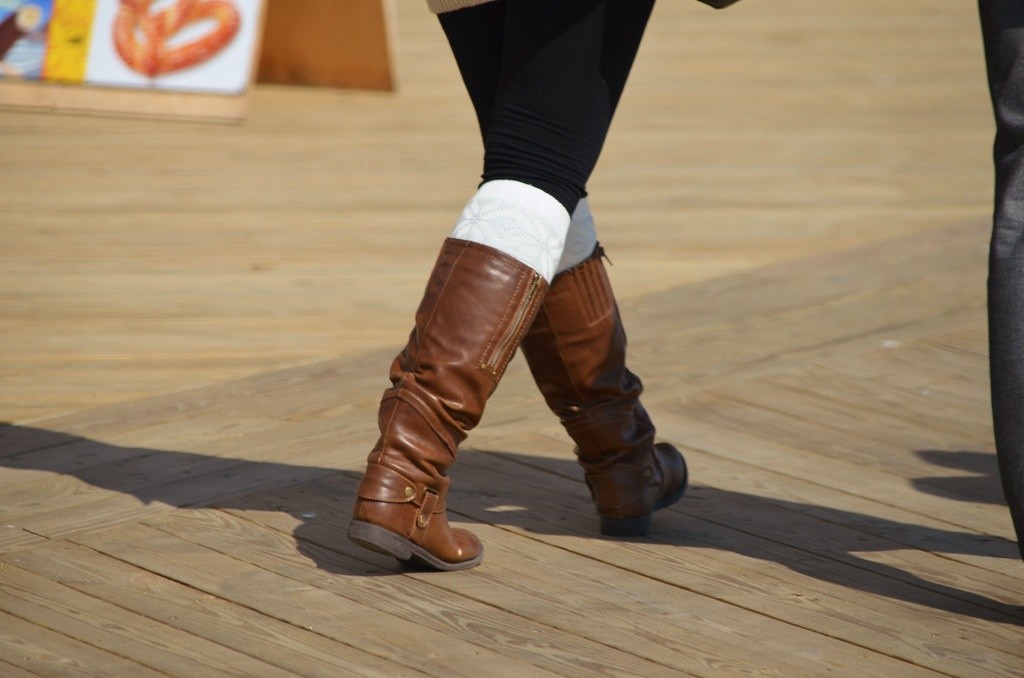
[346,237,552,571]
[518,242,689,537]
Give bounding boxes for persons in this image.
[978,0,1024,559]
[347,0,736,572]
